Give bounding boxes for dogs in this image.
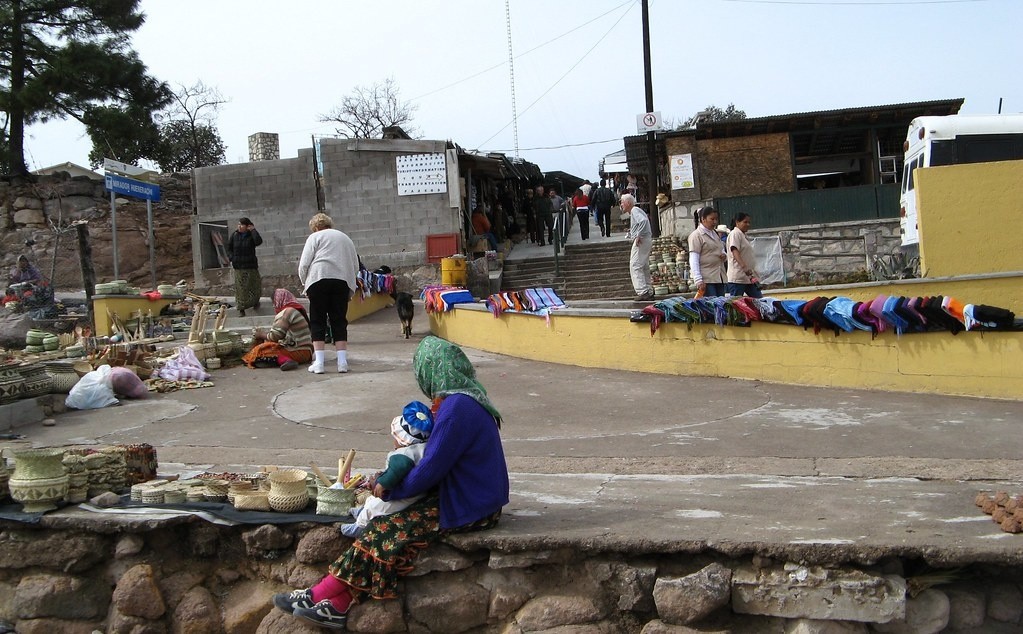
[390,292,414,339]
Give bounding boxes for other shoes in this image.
[341,524,362,538]
[253,302,259,310]
[350,508,360,519]
[280,360,298,371]
[634,292,655,301]
[238,311,245,317]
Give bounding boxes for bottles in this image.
[648,237,697,295]
[130,468,356,517]
[95,279,128,294]
[187,329,253,369]
[26,328,59,352]
[158,285,173,295]
[0,359,81,400]
[66,345,82,357]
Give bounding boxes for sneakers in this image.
[337,362,351,372]
[294,599,350,629]
[272,589,316,616]
[308,361,325,374]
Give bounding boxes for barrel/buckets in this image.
[440,258,467,287]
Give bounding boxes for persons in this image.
[298,212,359,375]
[2,255,42,308]
[572,180,616,240]
[688,206,730,298]
[521,186,564,247]
[620,194,655,301]
[274,336,510,629]
[253,288,315,371]
[227,217,263,318]
[472,186,510,253]
[726,212,763,298]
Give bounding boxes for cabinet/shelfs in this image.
[877,139,898,184]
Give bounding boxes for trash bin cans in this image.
[441,257,466,287]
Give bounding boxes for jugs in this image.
[0,446,69,514]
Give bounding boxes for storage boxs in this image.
[472,238,491,252]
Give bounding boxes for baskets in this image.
[0,443,157,514]
[203,467,356,517]
[0,360,93,405]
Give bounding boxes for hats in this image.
[391,400,433,445]
